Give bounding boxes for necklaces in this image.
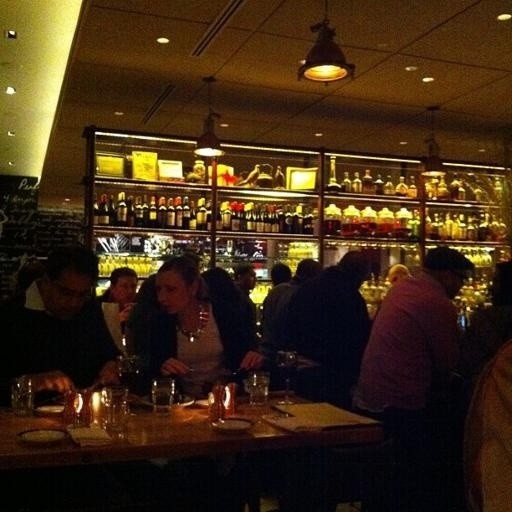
[179,304,209,342]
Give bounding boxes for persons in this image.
[387,263,412,286]
[261,258,324,342]
[231,260,258,326]
[351,246,475,430]
[128,252,210,340]
[98,267,138,359]
[140,256,265,397]
[1,244,124,406]
[467,260,512,374]
[270,262,292,286]
[277,250,373,405]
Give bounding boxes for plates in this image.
[140,394,194,410]
[211,417,254,432]
[17,428,71,445]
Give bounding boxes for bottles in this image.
[149,196,157,227]
[425,211,487,241]
[135,196,143,226]
[450,172,459,199]
[193,160,205,184]
[308,208,314,235]
[117,192,127,226]
[278,206,284,233]
[142,195,148,227]
[263,204,271,232]
[341,172,351,193]
[158,197,167,227]
[323,204,342,235]
[374,174,384,195]
[256,203,264,232]
[238,203,246,232]
[377,207,395,237]
[284,205,293,234]
[395,208,413,237]
[197,197,207,231]
[93,193,99,225]
[408,176,417,198]
[360,207,377,237]
[271,205,280,233]
[247,203,255,231]
[109,195,116,225]
[303,210,308,235]
[296,205,303,235]
[99,194,109,225]
[168,197,175,227]
[396,177,408,197]
[206,201,212,231]
[176,196,183,228]
[438,176,447,195]
[216,201,223,230]
[230,202,240,231]
[190,201,196,229]
[292,206,299,234]
[127,196,134,227]
[362,170,373,194]
[183,196,190,229]
[384,175,395,194]
[340,205,360,237]
[223,202,230,230]
[351,172,362,193]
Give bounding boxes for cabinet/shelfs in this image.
[78,124,512,326]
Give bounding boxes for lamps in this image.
[191,74,226,160]
[295,2,357,88]
[420,106,446,178]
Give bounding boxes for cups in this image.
[11,377,37,415]
[100,390,128,431]
[151,378,175,415]
[247,372,270,405]
